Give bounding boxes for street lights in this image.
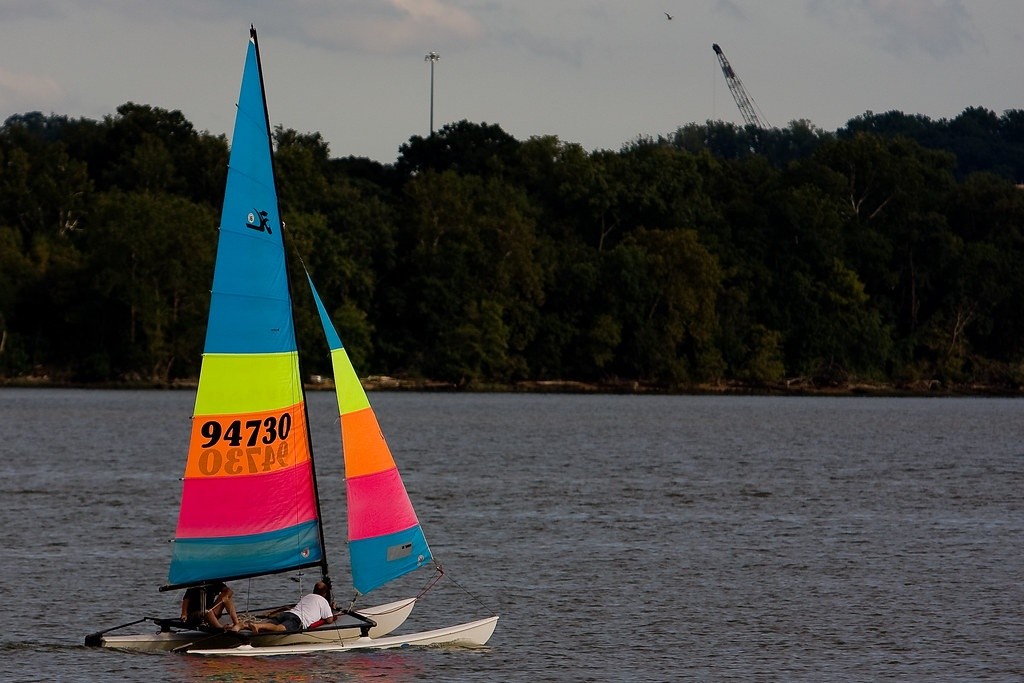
[424,50,440,134]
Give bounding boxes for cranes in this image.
[712,44,770,128]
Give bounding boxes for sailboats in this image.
[84,25,499,655]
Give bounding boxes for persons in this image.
[180,575,239,628]
[231,582,338,634]
